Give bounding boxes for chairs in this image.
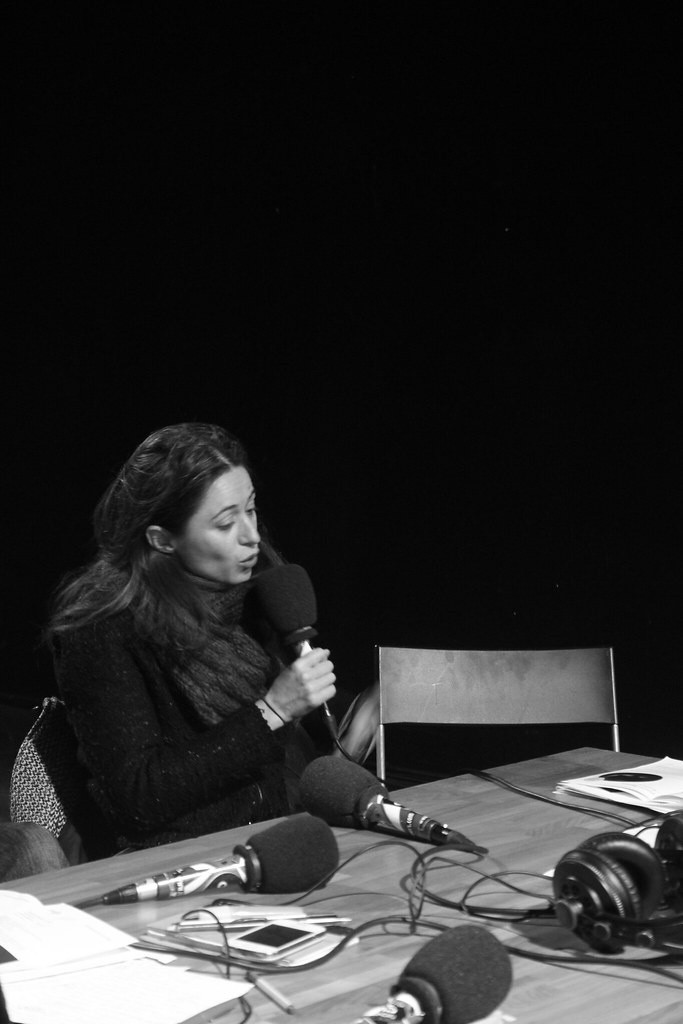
[369,645,618,791]
[7,693,120,873]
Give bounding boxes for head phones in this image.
[552,804,683,952]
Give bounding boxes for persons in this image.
[44,420,337,859]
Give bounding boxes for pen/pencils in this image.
[245,970,295,1015]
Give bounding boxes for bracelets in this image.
[261,697,289,725]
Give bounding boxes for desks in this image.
[1,745,683,1023]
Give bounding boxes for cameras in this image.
[222,920,328,962]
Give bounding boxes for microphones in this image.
[256,563,341,742]
[300,756,469,846]
[355,925,514,1024]
[102,814,339,905]
